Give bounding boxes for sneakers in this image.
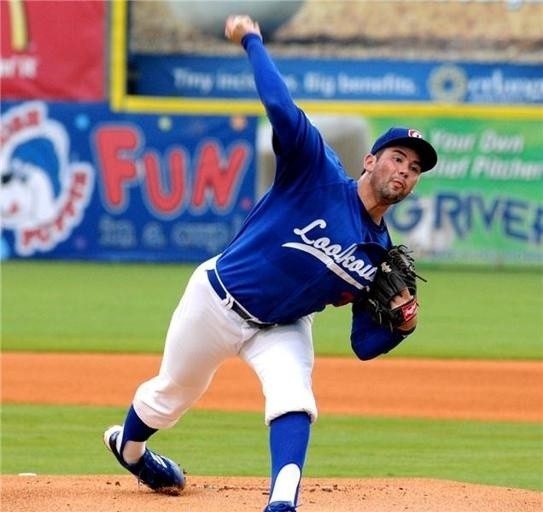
[263,501,302,512]
[102,424,187,491]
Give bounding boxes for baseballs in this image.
[232,15,248,29]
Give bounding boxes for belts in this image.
[207,270,275,329]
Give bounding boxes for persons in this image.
[103,15,438,512]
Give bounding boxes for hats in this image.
[370,127,437,173]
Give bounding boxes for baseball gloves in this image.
[367,248,418,327]
